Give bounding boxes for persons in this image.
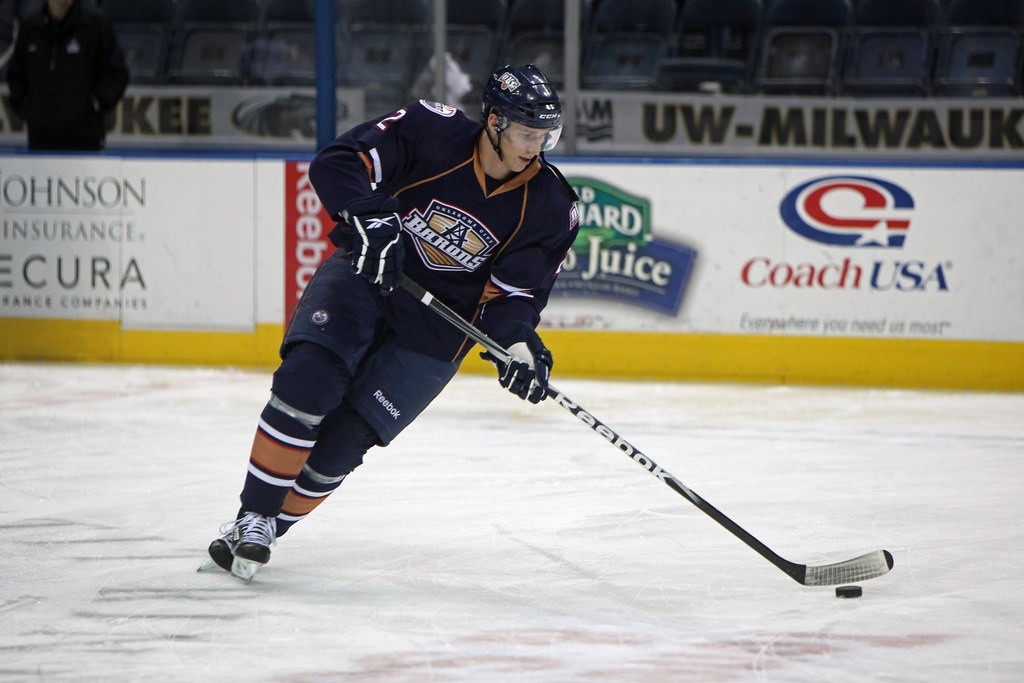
[7,0,129,150]
[196,62,580,585]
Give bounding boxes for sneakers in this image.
[209,506,277,570]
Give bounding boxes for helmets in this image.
[482,61,562,130]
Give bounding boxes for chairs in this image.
[80,0,1024,102]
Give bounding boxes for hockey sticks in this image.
[326,222,897,586]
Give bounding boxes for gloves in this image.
[326,190,408,296]
[479,318,554,404]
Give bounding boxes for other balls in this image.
[835,586,863,599]
